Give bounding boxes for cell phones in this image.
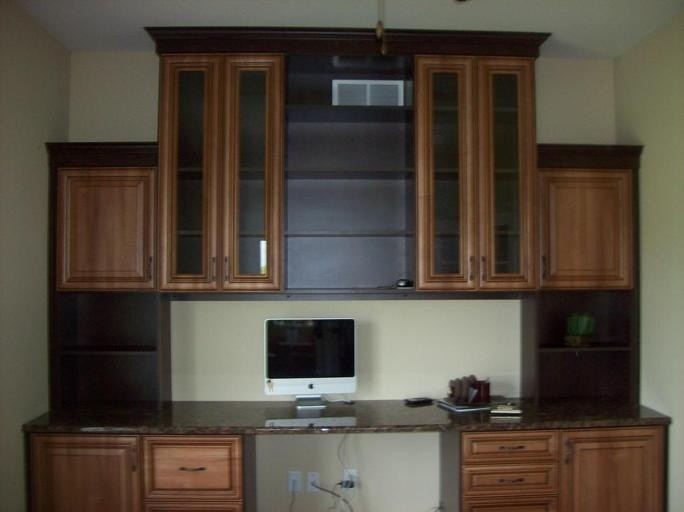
[405,396,431,406]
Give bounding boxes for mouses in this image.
[396,278,408,286]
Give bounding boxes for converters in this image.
[341,481,355,488]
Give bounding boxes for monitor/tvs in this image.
[263,318,357,410]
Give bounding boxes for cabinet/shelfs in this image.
[21,431,245,511]
[284,55,415,291]
[412,52,532,291]
[154,56,280,292]
[43,138,154,294]
[535,142,645,292]
[457,423,667,510]
